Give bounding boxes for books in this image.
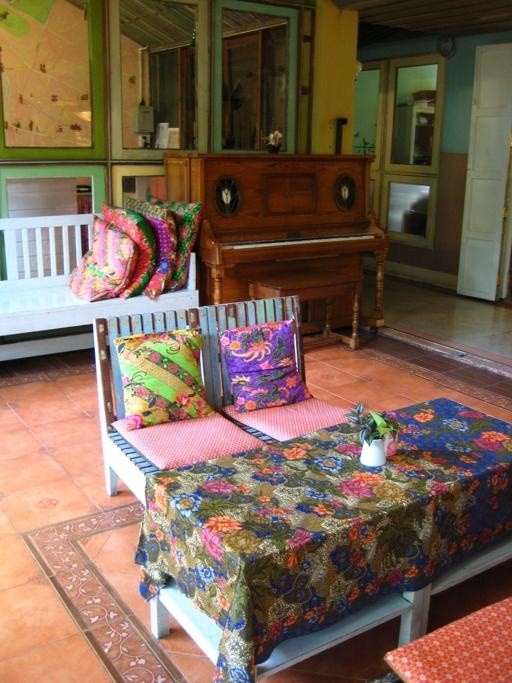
[153,120,180,148]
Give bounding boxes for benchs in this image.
[0,213,200,362]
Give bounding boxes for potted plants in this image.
[346,403,401,468]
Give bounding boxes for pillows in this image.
[66,195,206,303]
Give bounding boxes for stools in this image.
[247,272,363,351]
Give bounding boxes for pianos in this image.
[164,153,390,328]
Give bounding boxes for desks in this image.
[146,398,511,683]
